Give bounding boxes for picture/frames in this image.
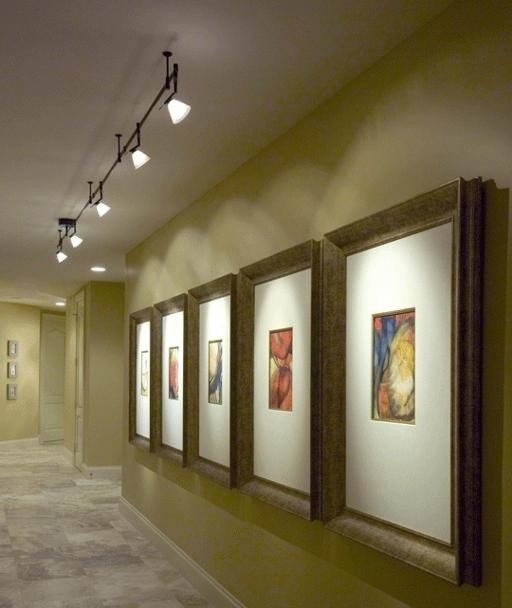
[186,272,234,491]
[234,238,318,522]
[6,339,19,401]
[317,175,484,589]
[152,293,186,469]
[128,305,152,456]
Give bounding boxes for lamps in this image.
[53,50,192,264]
